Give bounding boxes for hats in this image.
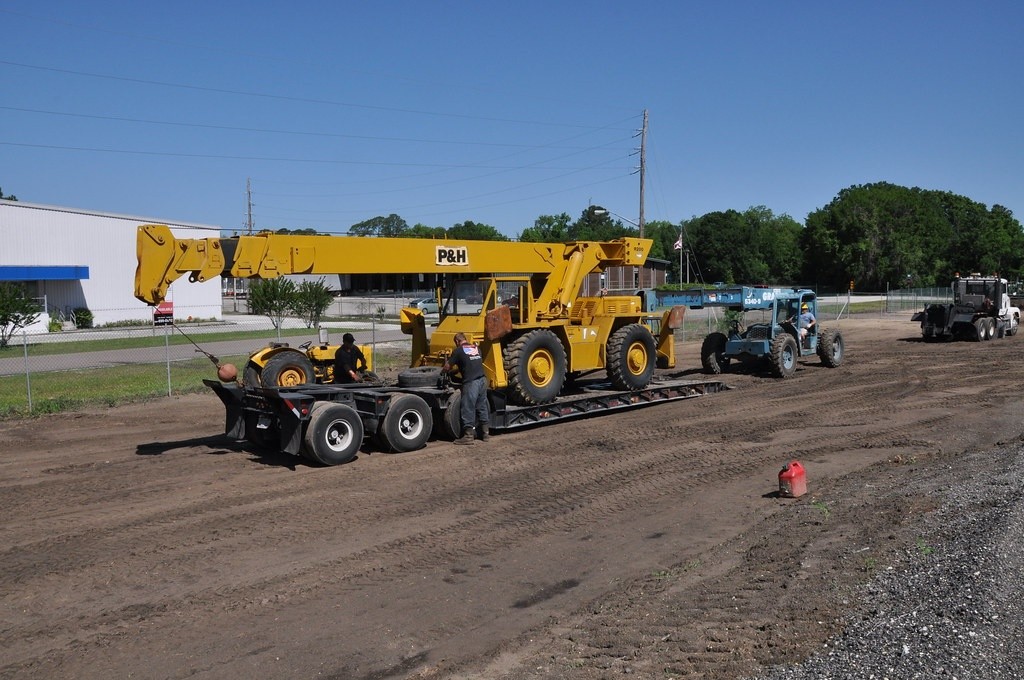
[343,333,356,341]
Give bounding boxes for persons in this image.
[782,303,816,346]
[332,333,368,385]
[440,332,491,446]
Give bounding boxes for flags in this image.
[673,232,682,250]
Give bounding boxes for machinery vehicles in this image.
[134,224,658,464]
[699,285,844,378]
[909,271,1021,343]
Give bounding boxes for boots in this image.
[454,428,474,445]
[478,424,490,442]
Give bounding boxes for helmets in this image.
[801,302,808,309]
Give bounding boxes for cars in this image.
[408,298,450,315]
[712,282,727,286]
